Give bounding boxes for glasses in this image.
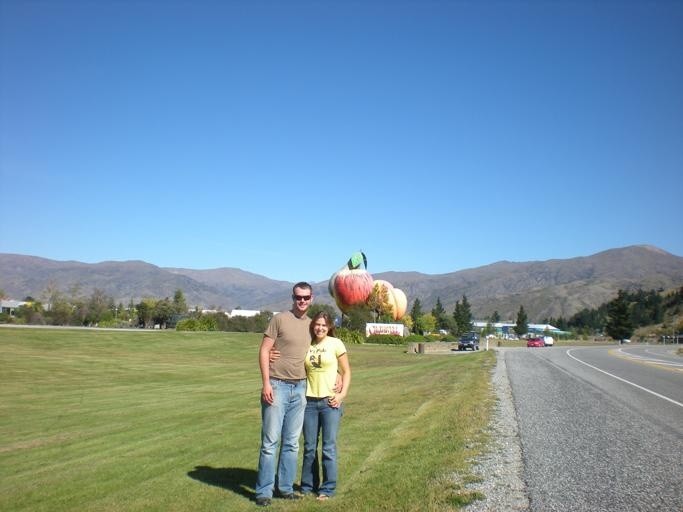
[294,294,311,300]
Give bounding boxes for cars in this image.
[485,334,495,339]
[527,338,545,347]
[504,333,518,341]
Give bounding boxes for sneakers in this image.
[255,498,273,507]
[316,492,331,502]
[298,490,315,496]
[275,492,302,500]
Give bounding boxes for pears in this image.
[329,250,361,297]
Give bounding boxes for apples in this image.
[327,269,374,307]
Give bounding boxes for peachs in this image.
[368,280,408,321]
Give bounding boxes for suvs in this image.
[540,336,553,346]
[459,331,480,351]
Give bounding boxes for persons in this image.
[267,309,351,501]
[254,280,344,507]
